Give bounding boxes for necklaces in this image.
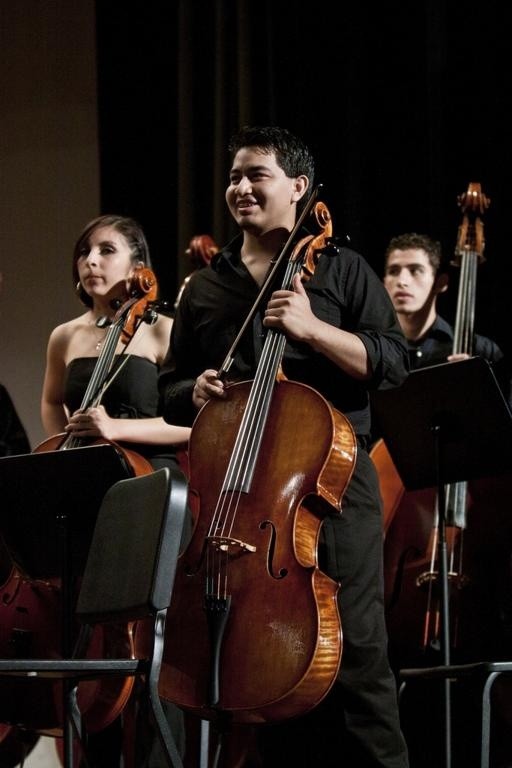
[88,317,107,352]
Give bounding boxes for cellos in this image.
[0,269,158,738]
[134,201,357,723]
[368,183,492,646]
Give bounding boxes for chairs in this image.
[395,562,512,767]
[1,462,188,767]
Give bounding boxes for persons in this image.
[0,383,31,457]
[157,123,414,768]
[382,231,512,411]
[40,211,193,768]
